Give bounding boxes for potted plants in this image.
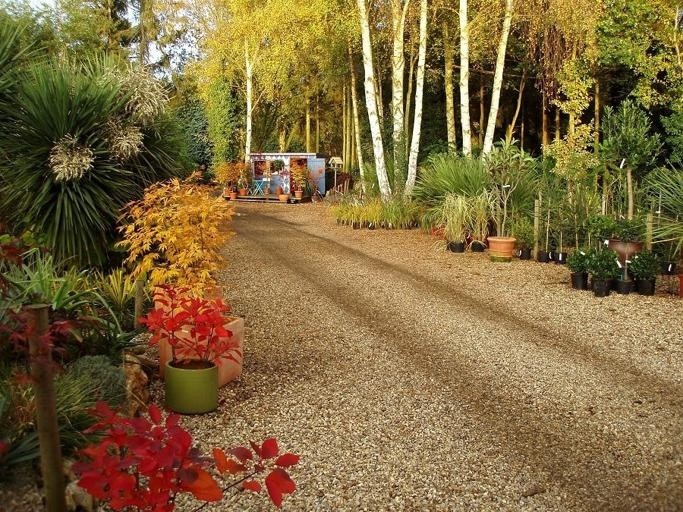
[443,193,492,255]
[631,249,656,297]
[612,256,631,297]
[587,247,615,297]
[568,248,590,290]
[138,274,235,418]
[587,214,647,264]
[480,175,519,262]
[211,157,310,204]
[121,173,247,392]
